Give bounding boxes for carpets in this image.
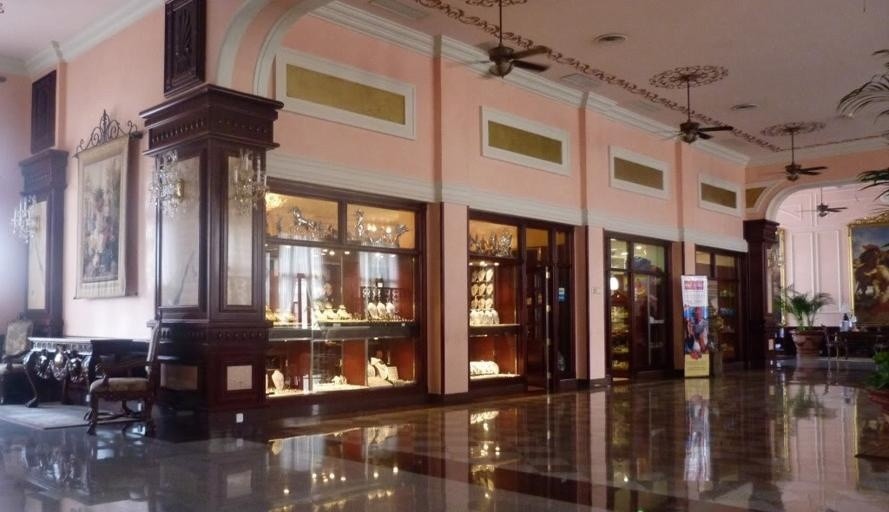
[0,399,122,431]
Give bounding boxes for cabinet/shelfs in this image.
[264,240,416,399]
[714,292,737,337]
[470,252,523,378]
[609,271,648,378]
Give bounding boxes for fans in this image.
[765,127,829,176]
[648,75,734,143]
[443,1,555,73]
[795,186,848,215]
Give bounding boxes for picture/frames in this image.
[846,208,889,329]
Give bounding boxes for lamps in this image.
[227,144,271,218]
[818,213,827,219]
[679,132,703,144]
[787,172,802,184]
[5,190,46,246]
[145,145,191,219]
[484,61,515,81]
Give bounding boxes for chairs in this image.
[81,318,163,437]
[1,316,38,406]
[821,323,888,360]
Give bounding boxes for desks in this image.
[20,335,134,421]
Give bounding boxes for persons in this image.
[683,305,710,361]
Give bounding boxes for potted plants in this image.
[770,280,839,360]
